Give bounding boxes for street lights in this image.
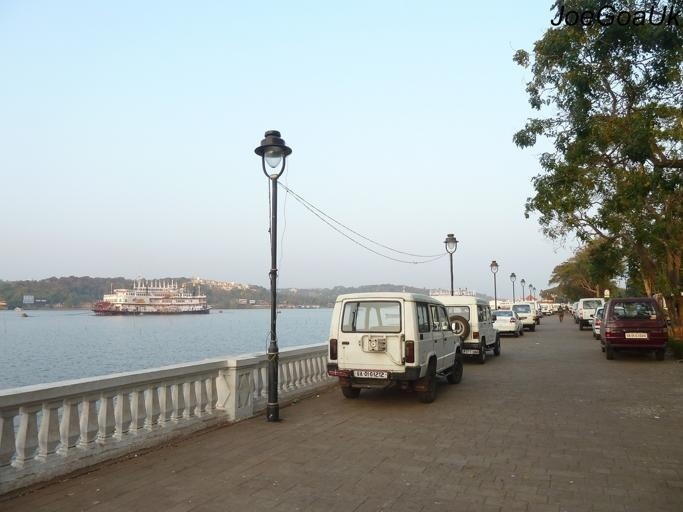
[488,261,500,310]
[443,234,459,295]
[521,279,536,301]
[509,273,516,303]
[254,130,291,421]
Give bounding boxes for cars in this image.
[491,310,523,337]
[511,301,568,331]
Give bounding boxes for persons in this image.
[558,306,564,323]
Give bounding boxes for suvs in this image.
[431,295,500,364]
[326,291,462,402]
[567,298,669,361]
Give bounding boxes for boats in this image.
[90,279,212,315]
